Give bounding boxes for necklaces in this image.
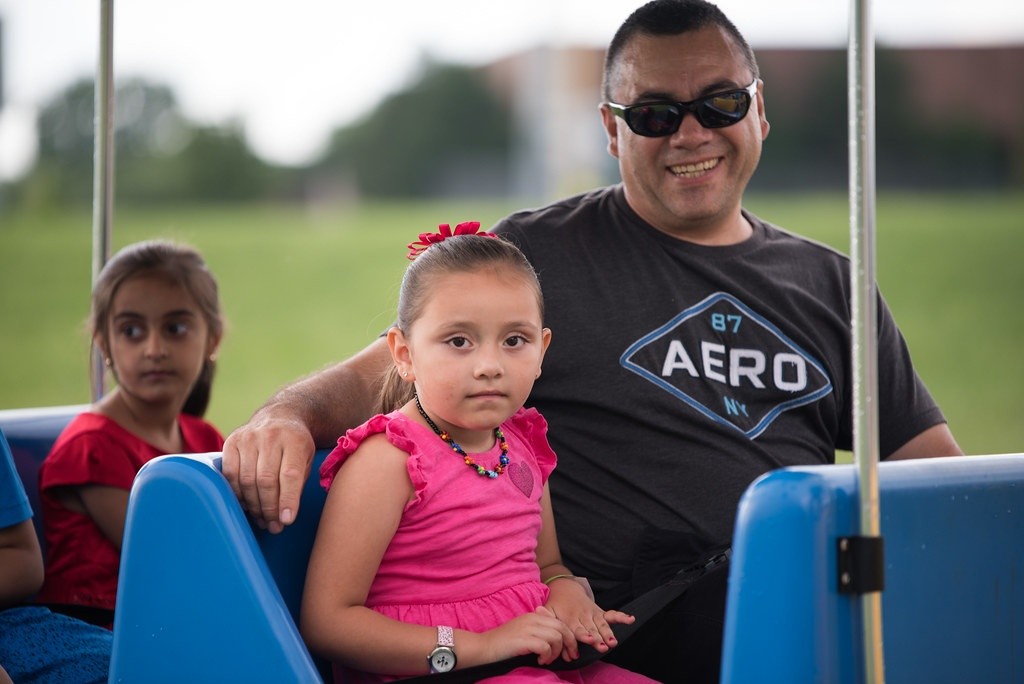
[414,393,510,479]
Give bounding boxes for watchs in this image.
[427,625,457,674]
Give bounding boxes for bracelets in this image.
[545,574,574,584]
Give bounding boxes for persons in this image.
[222,0,963,684]
[301,221,663,684]
[0,424,115,684]
[41,239,224,632]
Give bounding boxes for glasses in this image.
[606,77,759,138]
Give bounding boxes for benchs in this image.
[0,403,1024,684]
